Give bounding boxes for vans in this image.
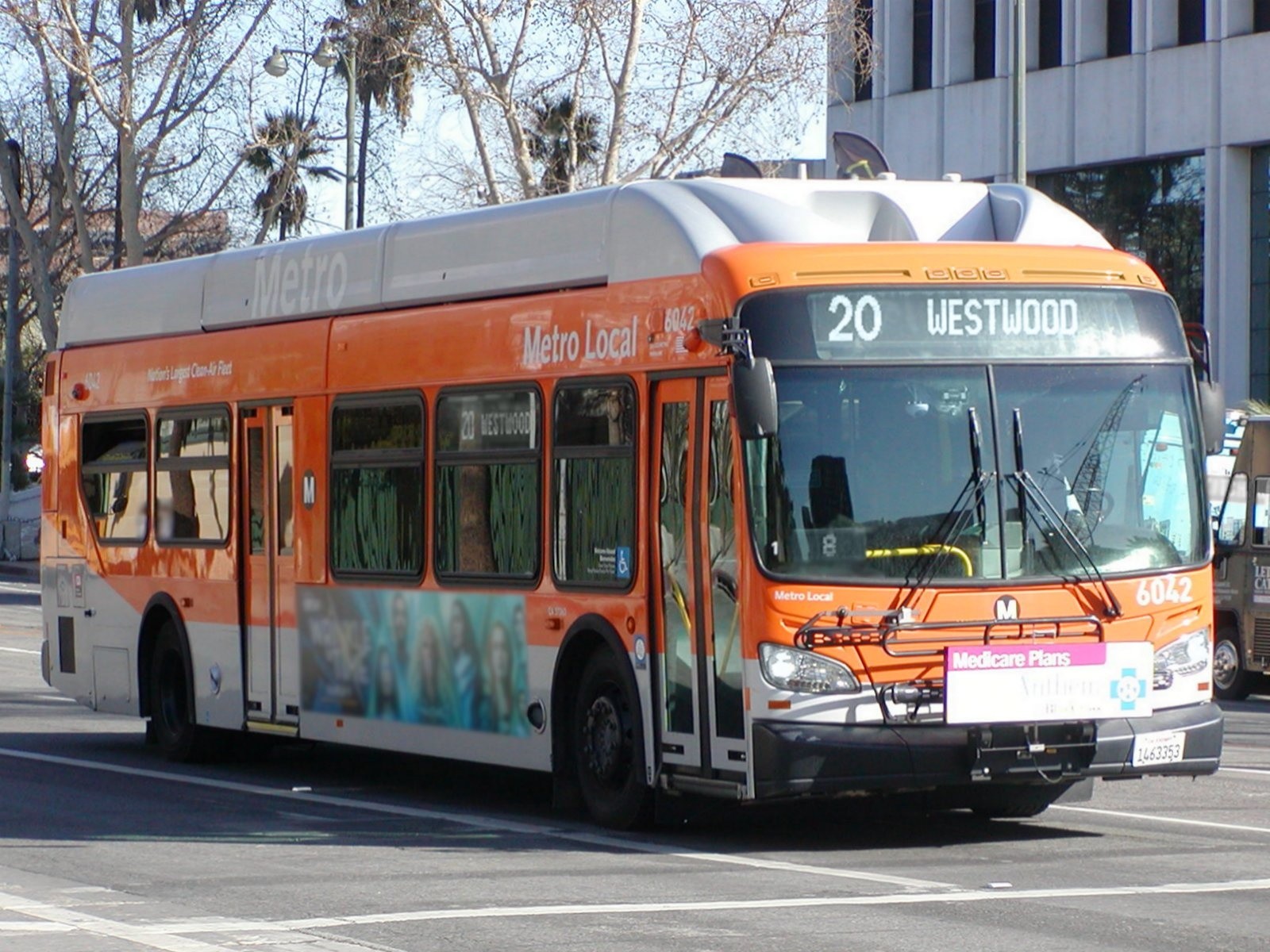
[1210,414,1270,701]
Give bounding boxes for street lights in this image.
[263,35,358,231]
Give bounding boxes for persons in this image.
[297,586,532,740]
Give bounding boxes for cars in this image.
[1204,452,1269,540]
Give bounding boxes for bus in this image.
[39,175,1226,839]
